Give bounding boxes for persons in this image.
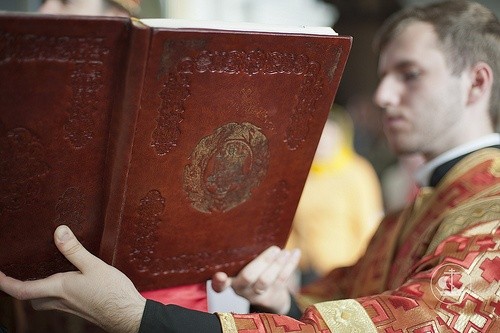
[34,0,140,15]
[283,22,384,285]
[0,0,500,333]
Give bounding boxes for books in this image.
[0,13,354,292]
